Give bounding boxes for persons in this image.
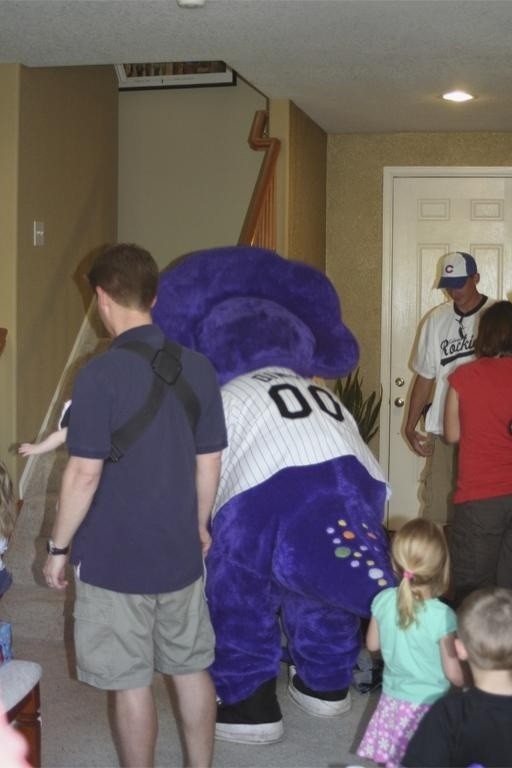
[43,242,228,768]
[355,517,471,768]
[445,300,511,609]
[401,586,512,768]
[405,252,498,524]
[18,427,68,457]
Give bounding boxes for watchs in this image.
[45,538,72,557]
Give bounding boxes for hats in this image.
[437,251,478,289]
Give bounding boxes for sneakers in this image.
[213,682,285,745]
[284,663,350,717]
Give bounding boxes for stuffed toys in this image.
[149,246,398,746]
